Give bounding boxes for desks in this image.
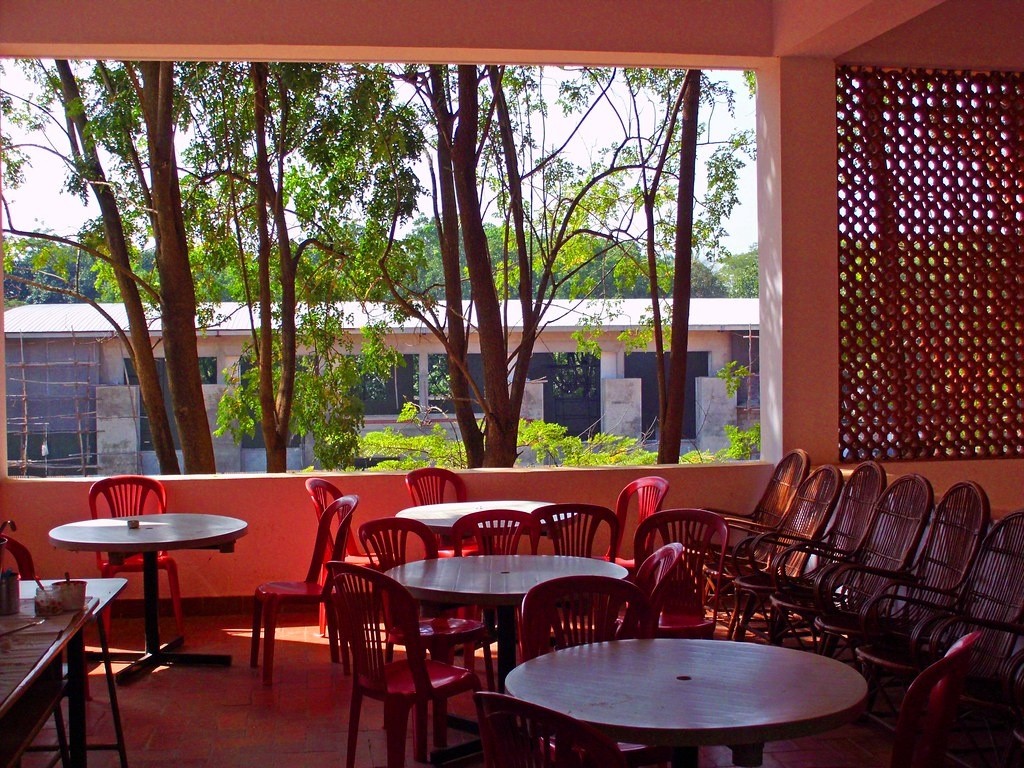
[48,513,248,686]
[504,637,869,768]
[0,578,128,768]
[383,554,629,694]
[394,500,584,554]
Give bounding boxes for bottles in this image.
[34,585,62,616]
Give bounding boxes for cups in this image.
[0,537,7,567]
[52,581,87,611]
[0,569,20,615]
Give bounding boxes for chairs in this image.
[251,467,729,768]
[697,449,1024,768]
[88,475,182,644]
